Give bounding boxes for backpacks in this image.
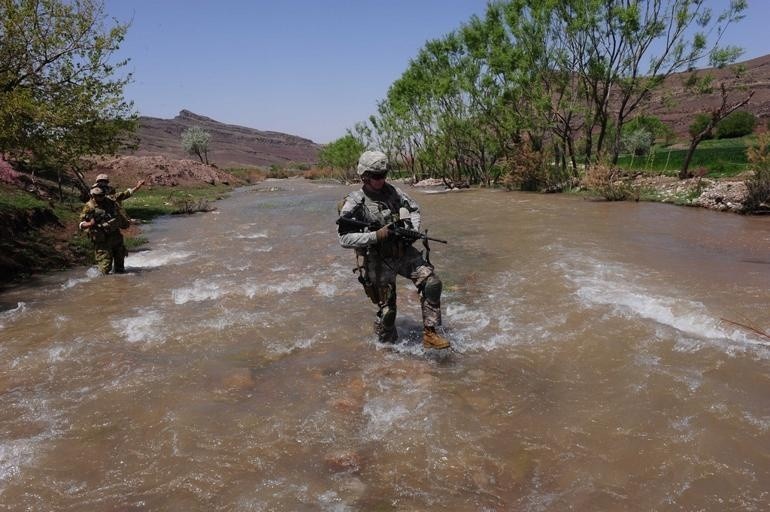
[338,192,364,217]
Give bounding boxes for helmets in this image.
[88,174,109,195]
[357,151,389,177]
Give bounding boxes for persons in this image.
[95,173,145,213]
[337,150,450,350]
[78,185,131,275]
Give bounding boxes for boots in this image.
[423,326,450,349]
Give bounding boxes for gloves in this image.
[377,222,402,247]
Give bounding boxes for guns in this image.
[71,166,93,199]
[336,216,448,243]
